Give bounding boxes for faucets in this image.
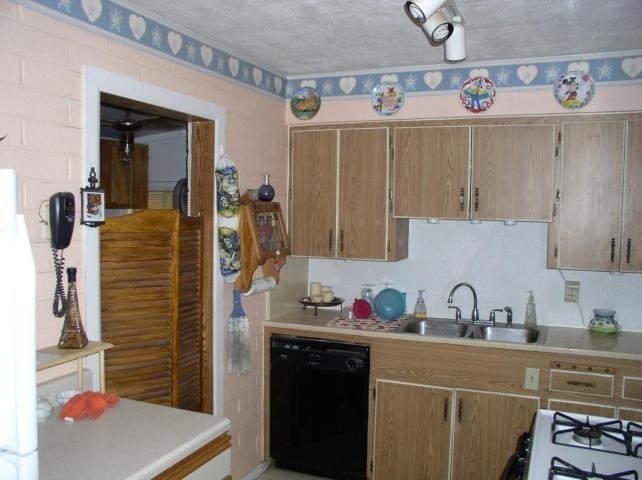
[448,284,478,320]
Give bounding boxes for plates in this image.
[290,86,321,120]
[458,74,498,114]
[553,70,598,110]
[370,80,405,116]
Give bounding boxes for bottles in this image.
[59,268,88,349]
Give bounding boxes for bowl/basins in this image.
[373,289,405,319]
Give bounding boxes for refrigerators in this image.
[0,169,41,479]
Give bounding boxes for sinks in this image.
[467,326,547,344]
[391,319,471,337]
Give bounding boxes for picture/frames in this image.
[80,167,105,228]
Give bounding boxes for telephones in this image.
[50,192,75,249]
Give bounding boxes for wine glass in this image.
[361,284,376,312]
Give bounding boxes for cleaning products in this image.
[414,290,427,319]
[525,290,538,327]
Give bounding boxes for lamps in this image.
[405,1,446,24]
[417,8,454,43]
[444,15,467,61]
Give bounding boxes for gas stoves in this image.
[521,408,642,480]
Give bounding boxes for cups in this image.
[309,282,334,304]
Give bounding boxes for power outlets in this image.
[563,280,580,303]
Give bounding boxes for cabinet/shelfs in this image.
[100,138,149,210]
[547,397,641,424]
[35,339,115,394]
[373,377,540,480]
[286,120,409,263]
[392,123,555,223]
[546,118,641,274]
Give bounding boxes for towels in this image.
[59,389,119,422]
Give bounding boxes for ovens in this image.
[271,333,370,479]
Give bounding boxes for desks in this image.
[37,391,232,480]
